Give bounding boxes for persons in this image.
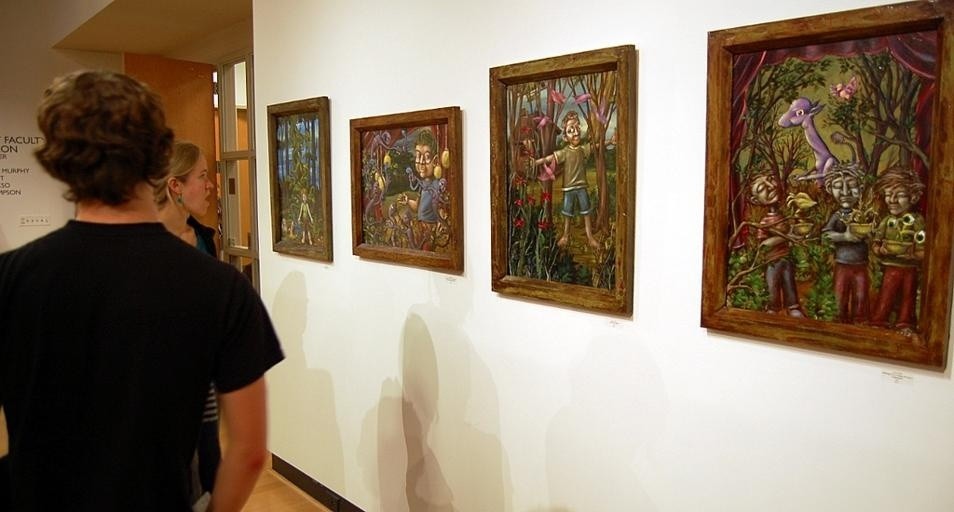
[153,140,222,496]
[822,161,870,324]
[397,128,440,251]
[522,110,617,248]
[2,68,287,512]
[297,187,315,246]
[745,168,806,318]
[872,168,926,332]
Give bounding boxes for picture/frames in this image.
[700,0,953,375]
[486,41,635,321]
[263,95,465,277]
